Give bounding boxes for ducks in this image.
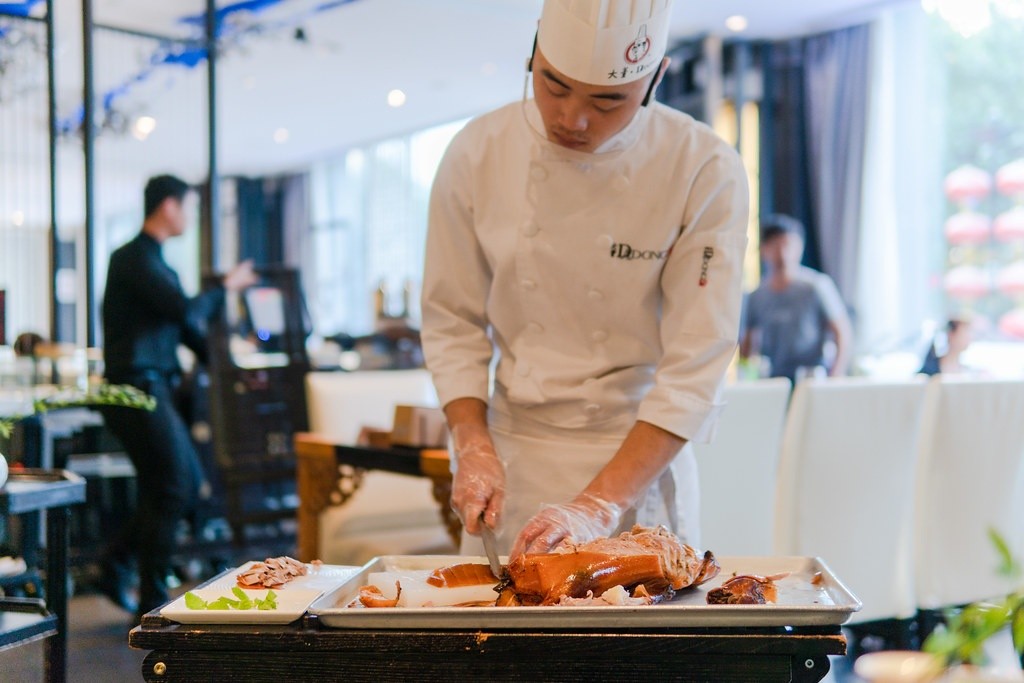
[492,524,785,605]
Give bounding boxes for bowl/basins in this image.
[853,650,968,683]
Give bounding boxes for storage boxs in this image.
[396,403,444,446]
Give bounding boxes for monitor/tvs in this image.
[242,285,285,338]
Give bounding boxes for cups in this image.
[792,365,826,383]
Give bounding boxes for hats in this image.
[537,0,671,85]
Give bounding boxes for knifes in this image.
[476,517,506,581]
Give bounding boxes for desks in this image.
[130,601,849,683]
[292,428,462,560]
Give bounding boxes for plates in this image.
[160,557,362,624]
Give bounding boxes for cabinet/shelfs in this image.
[0,468,87,683]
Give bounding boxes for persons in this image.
[724,216,850,391]
[922,317,984,378]
[423,0,749,578]
[102,174,260,621]
[14,334,76,365]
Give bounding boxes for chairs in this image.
[921,374,1022,633]
[782,376,920,647]
[693,375,793,557]
[306,368,437,437]
